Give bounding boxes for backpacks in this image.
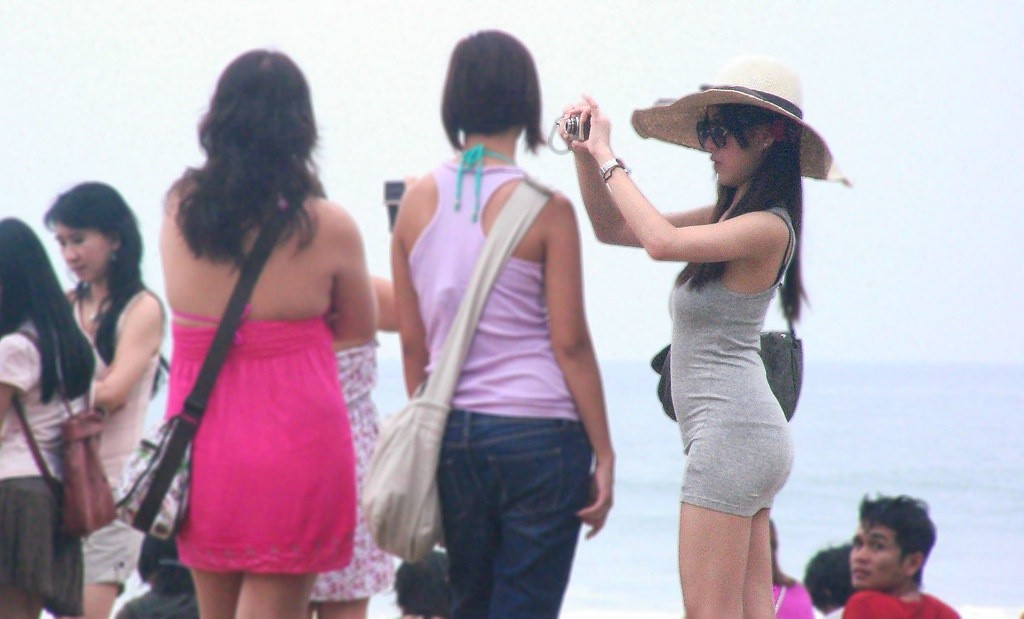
[11,330,119,536]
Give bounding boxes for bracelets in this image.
[604,166,628,182]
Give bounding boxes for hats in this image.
[631,62,832,180]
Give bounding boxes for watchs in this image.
[600,159,625,178]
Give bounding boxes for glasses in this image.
[696,118,769,149]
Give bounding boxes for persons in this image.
[557,57,850,618]
[113,536,201,618]
[1,182,166,619]
[161,49,378,619]
[394,551,450,619]
[311,277,396,618]
[769,495,962,619]
[390,30,617,619]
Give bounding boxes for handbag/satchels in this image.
[114,415,194,541]
[361,399,451,565]
[651,331,804,421]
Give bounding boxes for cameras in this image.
[563,115,590,142]
